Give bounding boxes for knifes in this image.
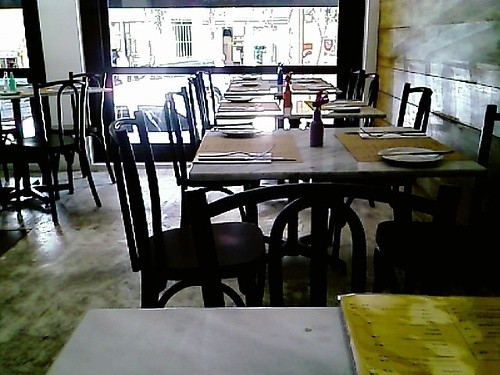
[378,150,455,157]
[198,156,297,162]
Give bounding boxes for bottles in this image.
[227,97,253,102]
[9,72,16,92]
[3,72,10,92]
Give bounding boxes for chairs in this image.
[0,69,500,308]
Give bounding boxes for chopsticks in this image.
[345,130,425,134]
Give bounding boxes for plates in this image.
[334,106,362,112]
[242,74,259,87]
[296,78,332,91]
[222,129,256,137]
[379,147,444,164]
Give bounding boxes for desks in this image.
[44,304,500,375]
[0,82,113,211]
[224,78,342,95]
[190,127,487,256]
[215,101,387,124]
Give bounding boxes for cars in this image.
[114,57,231,144]
